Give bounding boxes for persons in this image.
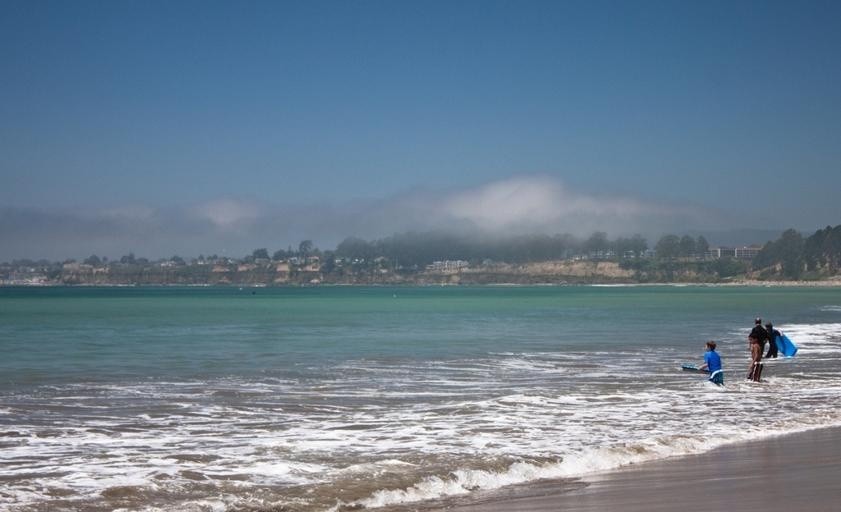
[746,317,786,383]
[697,340,723,388]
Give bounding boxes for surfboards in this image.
[682,362,712,372]
[773,329,797,356]
